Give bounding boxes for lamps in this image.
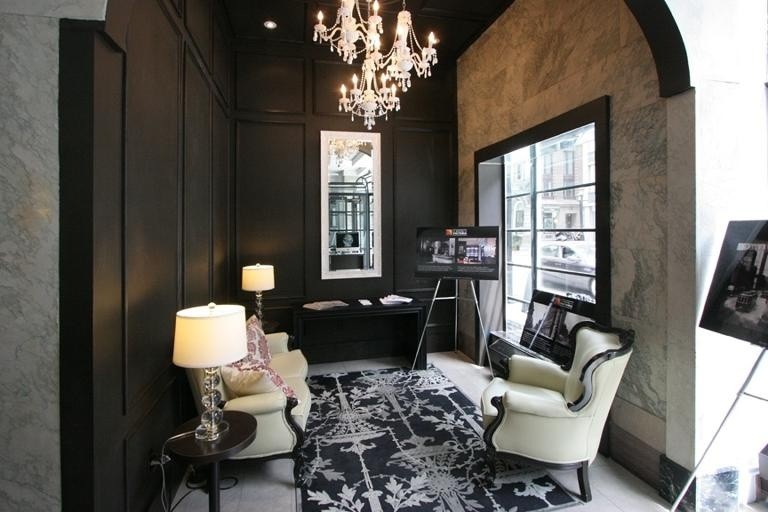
[308,0,440,130]
[328,137,365,168]
[241,264,275,317]
[173,302,248,440]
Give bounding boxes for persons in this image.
[553,233,584,262]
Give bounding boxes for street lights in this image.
[576,194,584,239]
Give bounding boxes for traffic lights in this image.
[551,207,561,225]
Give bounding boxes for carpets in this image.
[298,363,581,512]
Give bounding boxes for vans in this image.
[727,284,736,296]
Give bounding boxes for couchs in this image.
[481,321,636,502]
[186,331,314,488]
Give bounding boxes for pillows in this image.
[221,356,297,404]
[234,315,273,366]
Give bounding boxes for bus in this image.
[736,290,758,313]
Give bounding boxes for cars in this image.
[539,237,598,299]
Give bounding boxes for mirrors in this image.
[317,129,381,280]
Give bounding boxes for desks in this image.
[291,295,428,370]
[162,410,257,512]
[261,319,279,334]
